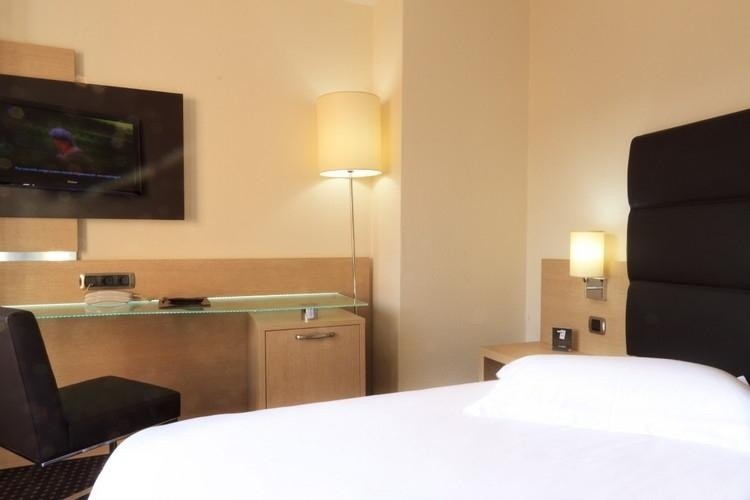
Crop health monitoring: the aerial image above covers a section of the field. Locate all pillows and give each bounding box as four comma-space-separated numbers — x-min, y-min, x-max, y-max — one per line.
495, 353, 749, 448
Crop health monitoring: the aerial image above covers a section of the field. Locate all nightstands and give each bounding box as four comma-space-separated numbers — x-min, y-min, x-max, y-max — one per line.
482, 258, 630, 383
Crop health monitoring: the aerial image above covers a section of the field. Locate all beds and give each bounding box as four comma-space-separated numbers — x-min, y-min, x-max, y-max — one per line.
88, 109, 750, 500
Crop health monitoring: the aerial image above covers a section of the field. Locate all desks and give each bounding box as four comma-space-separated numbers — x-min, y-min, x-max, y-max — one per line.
2, 293, 373, 415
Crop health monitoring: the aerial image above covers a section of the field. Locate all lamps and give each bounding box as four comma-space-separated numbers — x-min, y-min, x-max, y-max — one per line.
312, 89, 387, 317
568, 230, 608, 302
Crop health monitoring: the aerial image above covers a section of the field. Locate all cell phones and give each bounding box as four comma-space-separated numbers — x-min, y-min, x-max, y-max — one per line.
159, 296, 212, 311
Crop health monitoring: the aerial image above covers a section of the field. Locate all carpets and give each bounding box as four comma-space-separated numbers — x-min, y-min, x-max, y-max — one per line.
0, 454, 109, 499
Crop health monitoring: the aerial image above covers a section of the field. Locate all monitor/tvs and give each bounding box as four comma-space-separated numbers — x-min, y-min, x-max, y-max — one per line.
0, 73, 185, 220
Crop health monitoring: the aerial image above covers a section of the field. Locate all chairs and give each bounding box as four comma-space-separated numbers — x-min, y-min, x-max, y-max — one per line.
0, 308, 183, 500
